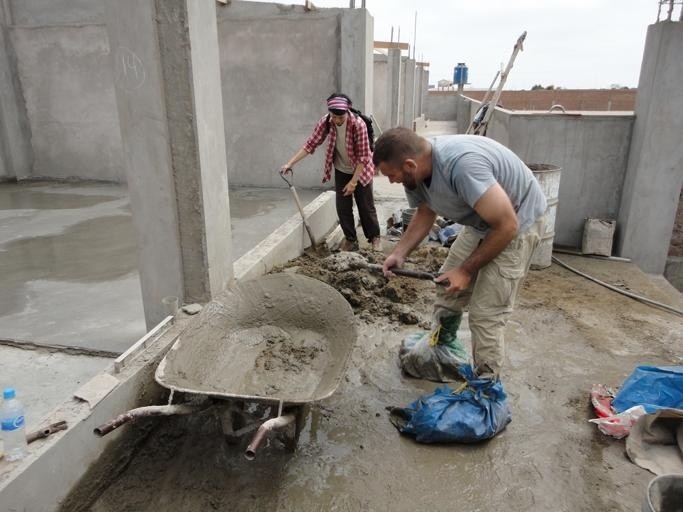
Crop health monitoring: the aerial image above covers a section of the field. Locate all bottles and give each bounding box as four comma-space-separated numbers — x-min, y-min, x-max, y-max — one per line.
0, 387, 30, 461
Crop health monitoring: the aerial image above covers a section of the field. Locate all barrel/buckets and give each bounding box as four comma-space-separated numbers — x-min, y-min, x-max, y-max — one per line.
582, 217, 617, 258
528, 162, 563, 271
401, 209, 428, 248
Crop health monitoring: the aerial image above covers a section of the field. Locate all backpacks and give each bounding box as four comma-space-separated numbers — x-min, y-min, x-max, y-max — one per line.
350, 108, 374, 152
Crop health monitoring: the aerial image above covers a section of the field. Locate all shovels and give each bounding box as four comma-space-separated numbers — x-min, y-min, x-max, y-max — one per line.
327, 251, 451, 287
278, 169, 331, 258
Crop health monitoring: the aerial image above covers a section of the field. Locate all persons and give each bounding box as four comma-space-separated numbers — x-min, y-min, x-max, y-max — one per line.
370, 124, 547, 386
278, 92, 382, 253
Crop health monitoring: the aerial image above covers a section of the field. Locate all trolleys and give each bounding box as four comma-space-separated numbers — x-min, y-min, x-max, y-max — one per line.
90, 268, 361, 464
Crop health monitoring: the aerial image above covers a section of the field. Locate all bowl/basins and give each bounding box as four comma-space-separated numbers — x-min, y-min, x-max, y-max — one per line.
591, 388, 630, 432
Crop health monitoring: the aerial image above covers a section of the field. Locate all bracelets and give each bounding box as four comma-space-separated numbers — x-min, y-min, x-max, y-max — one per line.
349, 181, 357, 187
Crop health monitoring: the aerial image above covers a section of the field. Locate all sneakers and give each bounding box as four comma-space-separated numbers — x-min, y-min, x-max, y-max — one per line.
371, 238, 383, 251
344, 240, 359, 250
